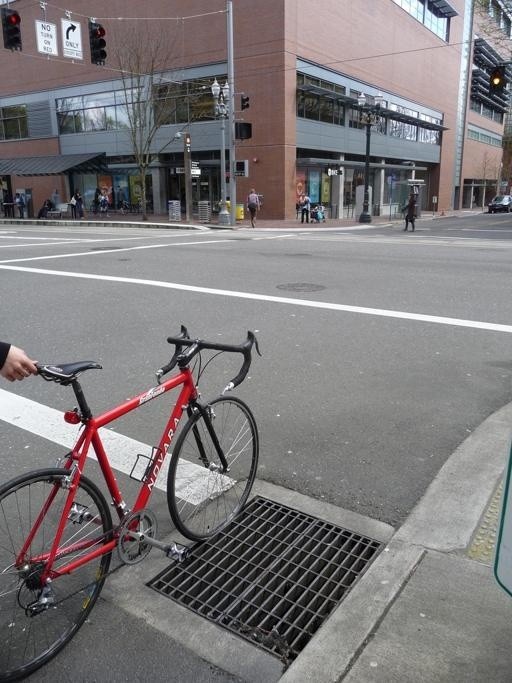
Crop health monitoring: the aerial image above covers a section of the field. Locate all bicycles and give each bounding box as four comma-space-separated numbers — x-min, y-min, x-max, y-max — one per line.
1, 326, 264, 681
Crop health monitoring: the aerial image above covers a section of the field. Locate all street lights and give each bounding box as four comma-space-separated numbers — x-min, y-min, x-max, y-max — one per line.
356, 93, 384, 223
210, 78, 230, 224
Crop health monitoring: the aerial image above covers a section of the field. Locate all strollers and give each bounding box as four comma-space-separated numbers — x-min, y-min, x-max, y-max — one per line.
310, 205, 326, 223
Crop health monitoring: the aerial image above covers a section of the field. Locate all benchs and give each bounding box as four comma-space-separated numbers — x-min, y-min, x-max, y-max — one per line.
35, 202, 71, 219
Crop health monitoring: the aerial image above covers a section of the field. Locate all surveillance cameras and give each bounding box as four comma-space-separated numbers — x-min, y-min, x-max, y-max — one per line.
175, 133, 181, 139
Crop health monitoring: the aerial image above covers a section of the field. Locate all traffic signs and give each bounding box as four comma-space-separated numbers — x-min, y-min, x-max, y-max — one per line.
60, 17, 84, 60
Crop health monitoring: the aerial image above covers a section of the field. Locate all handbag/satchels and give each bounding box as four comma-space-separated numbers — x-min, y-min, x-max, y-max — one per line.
248, 203, 257, 212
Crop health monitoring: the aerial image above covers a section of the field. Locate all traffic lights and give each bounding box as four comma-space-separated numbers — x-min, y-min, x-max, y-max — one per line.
89, 19, 107, 65
0, 7, 20, 46
490, 66, 505, 91
241, 95, 250, 111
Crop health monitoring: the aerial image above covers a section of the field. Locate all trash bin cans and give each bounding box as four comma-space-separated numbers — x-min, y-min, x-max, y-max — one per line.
197, 200, 211, 224
168, 200, 182, 222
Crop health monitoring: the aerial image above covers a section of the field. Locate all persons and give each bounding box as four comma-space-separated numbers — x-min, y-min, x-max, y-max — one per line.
246, 188, 260, 229
299, 193, 311, 224
401, 192, 417, 232
0, 342, 38, 382
3, 187, 126, 219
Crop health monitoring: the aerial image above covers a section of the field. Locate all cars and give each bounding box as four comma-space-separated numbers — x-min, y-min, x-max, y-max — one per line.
488, 195, 512, 212
